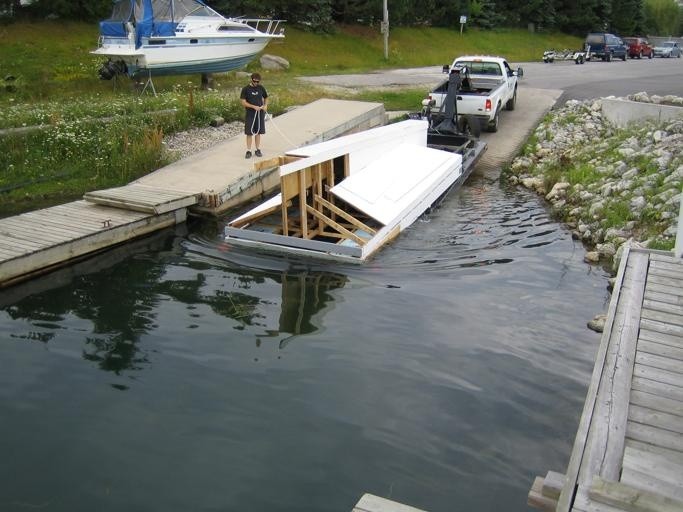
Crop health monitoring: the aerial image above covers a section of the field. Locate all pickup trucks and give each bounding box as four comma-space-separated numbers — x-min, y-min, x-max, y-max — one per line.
418, 54, 523, 132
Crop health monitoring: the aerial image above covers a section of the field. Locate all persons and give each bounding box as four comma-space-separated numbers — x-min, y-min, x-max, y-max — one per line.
239, 71, 270, 160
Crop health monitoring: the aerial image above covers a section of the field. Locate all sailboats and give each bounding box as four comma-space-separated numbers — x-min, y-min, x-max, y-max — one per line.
90, 0, 289, 81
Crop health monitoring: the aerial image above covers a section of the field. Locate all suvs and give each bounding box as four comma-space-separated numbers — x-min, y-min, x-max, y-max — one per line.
584, 33, 628, 61
621, 37, 654, 60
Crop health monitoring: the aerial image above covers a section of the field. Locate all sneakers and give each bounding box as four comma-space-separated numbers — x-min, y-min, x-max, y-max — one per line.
246, 151, 252, 158
255, 149, 262, 157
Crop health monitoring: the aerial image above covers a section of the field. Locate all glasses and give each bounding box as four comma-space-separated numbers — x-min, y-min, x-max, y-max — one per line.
252, 80, 260, 83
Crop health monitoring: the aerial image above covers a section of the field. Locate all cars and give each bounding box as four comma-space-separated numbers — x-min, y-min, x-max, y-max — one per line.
653, 41, 681, 58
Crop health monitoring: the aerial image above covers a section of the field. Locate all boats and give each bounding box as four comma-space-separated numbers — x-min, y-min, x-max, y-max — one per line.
216, 113, 486, 269
